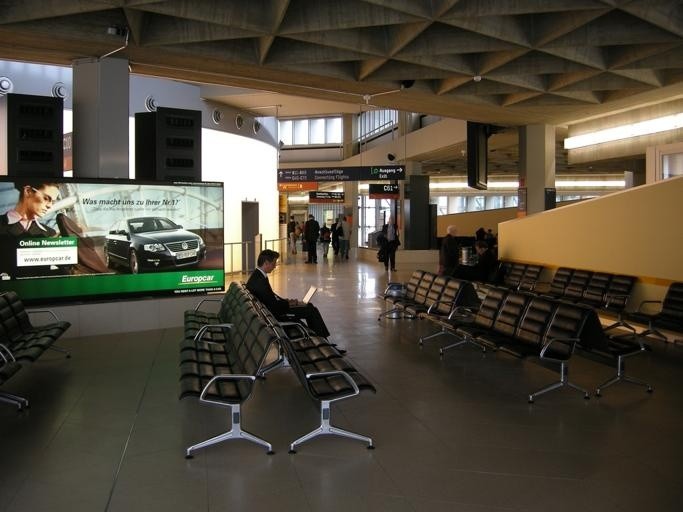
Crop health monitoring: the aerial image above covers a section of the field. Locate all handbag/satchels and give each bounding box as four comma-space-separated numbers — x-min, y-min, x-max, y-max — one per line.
336, 221, 343, 236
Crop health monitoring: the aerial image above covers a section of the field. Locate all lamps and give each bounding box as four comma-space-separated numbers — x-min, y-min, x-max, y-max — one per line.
562, 110, 683, 150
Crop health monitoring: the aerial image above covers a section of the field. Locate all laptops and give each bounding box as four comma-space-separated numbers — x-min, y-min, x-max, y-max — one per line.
291, 285, 317, 307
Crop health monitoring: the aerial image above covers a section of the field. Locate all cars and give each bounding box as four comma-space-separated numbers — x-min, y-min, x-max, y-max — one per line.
103, 216, 208, 273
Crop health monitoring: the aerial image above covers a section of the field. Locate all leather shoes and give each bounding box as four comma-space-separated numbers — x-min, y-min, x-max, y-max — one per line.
390, 268, 397, 272
385, 267, 388, 272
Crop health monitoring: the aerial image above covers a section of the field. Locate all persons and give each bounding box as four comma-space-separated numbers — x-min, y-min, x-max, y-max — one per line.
0, 182, 76, 277
300, 222, 305, 239
320, 222, 332, 257
454, 240, 496, 282
304, 215, 320, 264
297, 221, 301, 239
288, 216, 299, 254
476, 228, 486, 239
336, 215, 352, 259
330, 218, 345, 255
381, 217, 401, 272
438, 225, 459, 277
245, 250, 348, 354
487, 229, 496, 239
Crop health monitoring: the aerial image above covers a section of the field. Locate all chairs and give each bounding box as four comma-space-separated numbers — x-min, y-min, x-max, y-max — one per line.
182, 281, 250, 341
253, 300, 379, 457
238, 280, 316, 339
0, 290, 73, 367
0, 350, 23, 412
484, 283, 653, 396
627, 280, 683, 345
539, 265, 642, 343
177, 300, 284, 460
376, 269, 467, 347
496, 258, 544, 292
